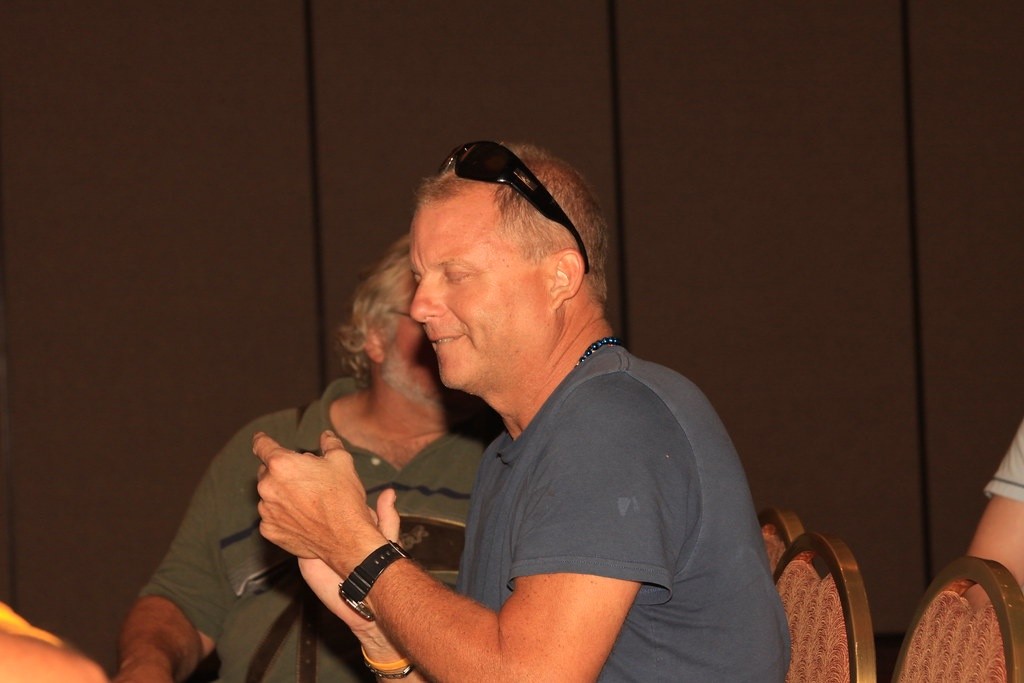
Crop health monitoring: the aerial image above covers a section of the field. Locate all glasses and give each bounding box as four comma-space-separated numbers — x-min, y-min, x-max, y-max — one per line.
437, 141, 591, 276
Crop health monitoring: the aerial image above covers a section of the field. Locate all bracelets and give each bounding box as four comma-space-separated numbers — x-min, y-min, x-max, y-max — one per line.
361, 644, 411, 669
370, 665, 415, 679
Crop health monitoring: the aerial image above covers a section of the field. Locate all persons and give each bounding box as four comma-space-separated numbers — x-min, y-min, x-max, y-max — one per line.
962, 421, 1024, 612
104, 235, 494, 683
0, 601, 110, 683
252, 143, 791, 683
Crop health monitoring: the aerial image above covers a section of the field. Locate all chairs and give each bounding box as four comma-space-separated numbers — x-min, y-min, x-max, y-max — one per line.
755, 504, 1024, 683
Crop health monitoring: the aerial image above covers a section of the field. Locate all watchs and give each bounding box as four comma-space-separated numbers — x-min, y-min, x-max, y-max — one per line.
337, 540, 411, 619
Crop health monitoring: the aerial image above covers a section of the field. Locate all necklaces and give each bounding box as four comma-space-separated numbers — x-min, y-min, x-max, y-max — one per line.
580, 337, 621, 363
